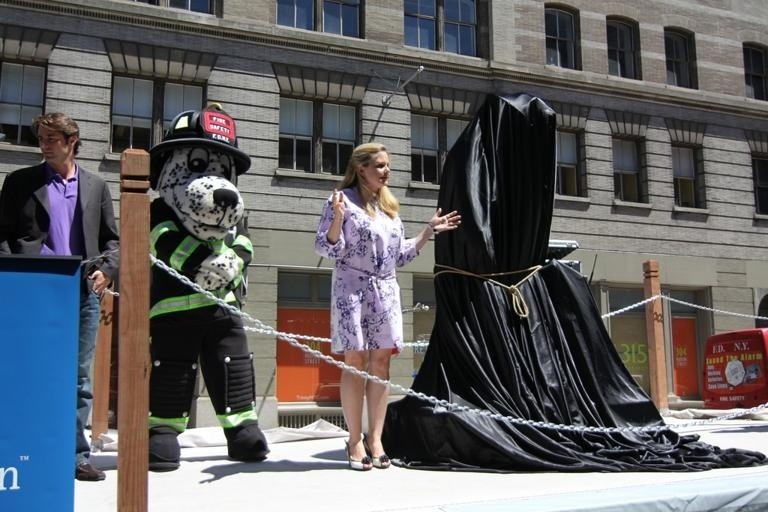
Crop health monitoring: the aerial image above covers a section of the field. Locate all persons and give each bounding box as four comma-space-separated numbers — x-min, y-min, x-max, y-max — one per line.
315, 143, 464, 471
2, 111, 119, 481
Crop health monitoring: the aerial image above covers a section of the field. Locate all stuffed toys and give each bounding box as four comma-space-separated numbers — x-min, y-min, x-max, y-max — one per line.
115, 101, 270, 472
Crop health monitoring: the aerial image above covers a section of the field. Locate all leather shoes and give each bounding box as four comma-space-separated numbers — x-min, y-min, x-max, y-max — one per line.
76, 464, 106, 482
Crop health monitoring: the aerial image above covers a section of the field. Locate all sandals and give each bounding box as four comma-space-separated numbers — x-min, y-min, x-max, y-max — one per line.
363, 436, 391, 469
343, 441, 372, 471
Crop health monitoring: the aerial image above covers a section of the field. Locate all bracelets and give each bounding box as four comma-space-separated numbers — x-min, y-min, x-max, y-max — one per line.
428, 223, 436, 234
